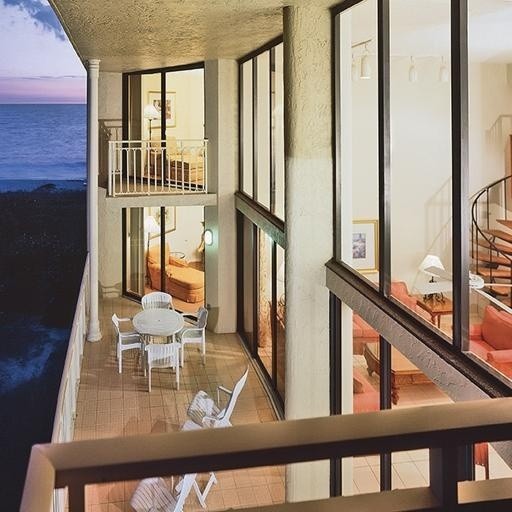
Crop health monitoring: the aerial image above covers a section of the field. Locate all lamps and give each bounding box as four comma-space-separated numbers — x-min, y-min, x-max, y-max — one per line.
419, 255, 446, 303
359, 41, 377, 79
146, 216, 160, 284
439, 57, 451, 83
407, 56, 419, 81
144, 104, 160, 175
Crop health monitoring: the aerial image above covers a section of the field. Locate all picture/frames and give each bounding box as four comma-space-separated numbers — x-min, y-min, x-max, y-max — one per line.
147, 90, 179, 130
352, 220, 380, 275
148, 206, 177, 240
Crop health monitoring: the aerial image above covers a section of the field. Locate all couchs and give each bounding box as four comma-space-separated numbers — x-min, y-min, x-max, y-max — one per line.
351, 280, 418, 354
353, 368, 381, 414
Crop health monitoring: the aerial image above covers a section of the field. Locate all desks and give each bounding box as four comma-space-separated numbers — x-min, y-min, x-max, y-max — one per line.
364, 342, 434, 405
414, 296, 454, 328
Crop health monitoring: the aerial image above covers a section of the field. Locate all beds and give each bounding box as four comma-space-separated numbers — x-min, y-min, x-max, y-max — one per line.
146, 135, 204, 183
145, 242, 204, 303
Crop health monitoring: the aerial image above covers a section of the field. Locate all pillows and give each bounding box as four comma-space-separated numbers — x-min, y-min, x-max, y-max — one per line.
353, 376, 362, 392
480, 305, 512, 350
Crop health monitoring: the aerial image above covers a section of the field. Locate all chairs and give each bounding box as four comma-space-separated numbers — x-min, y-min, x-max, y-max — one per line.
111, 291, 208, 393
452, 302, 512, 380
128, 364, 250, 512
